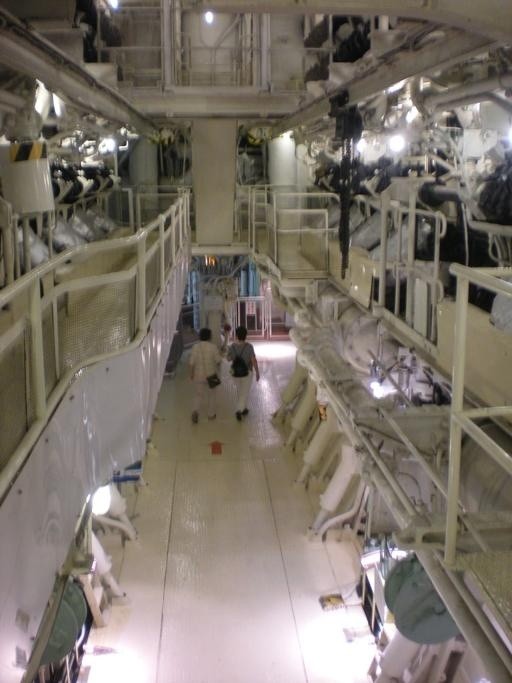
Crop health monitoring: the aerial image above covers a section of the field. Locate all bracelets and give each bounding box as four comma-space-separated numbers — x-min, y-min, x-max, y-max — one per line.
255, 367, 258, 370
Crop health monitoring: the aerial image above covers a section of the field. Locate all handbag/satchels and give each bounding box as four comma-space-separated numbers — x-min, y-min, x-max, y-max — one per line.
206, 374, 221, 388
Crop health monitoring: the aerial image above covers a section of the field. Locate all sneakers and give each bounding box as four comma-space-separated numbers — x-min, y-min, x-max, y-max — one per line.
235, 408, 248, 421
208, 414, 216, 421
192, 411, 198, 424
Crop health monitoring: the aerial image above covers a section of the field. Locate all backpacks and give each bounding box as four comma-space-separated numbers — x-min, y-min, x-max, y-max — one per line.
231, 356, 249, 377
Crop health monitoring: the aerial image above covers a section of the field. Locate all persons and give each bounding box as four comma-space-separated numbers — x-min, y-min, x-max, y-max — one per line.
187, 328, 222, 423
225, 325, 260, 421
